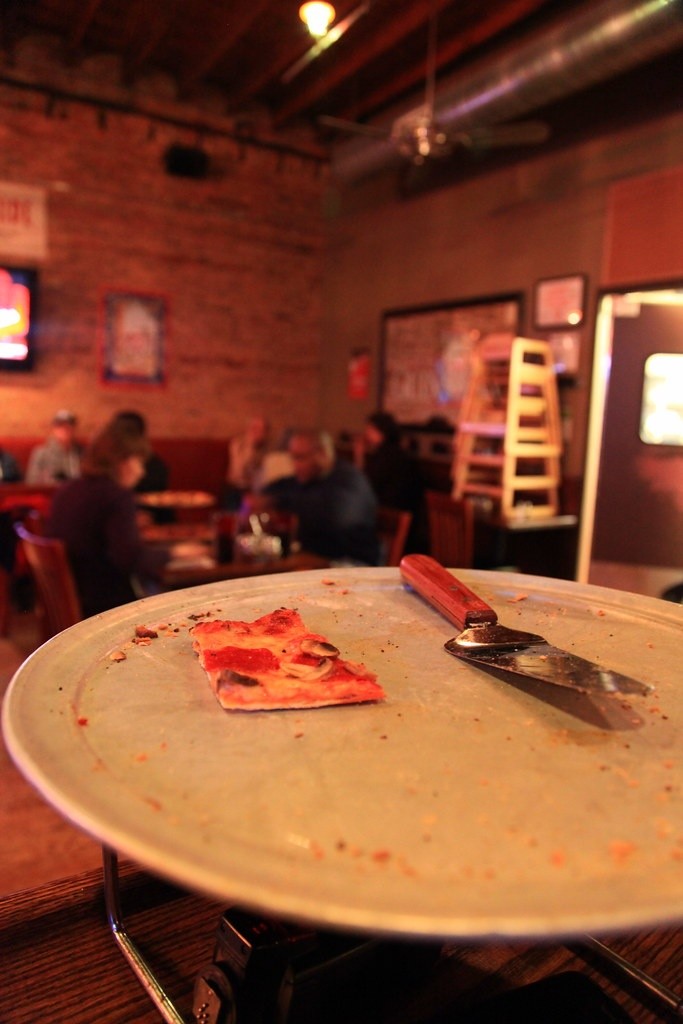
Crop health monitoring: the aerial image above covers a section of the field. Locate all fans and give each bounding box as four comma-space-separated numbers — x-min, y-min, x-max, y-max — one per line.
322, 0, 552, 199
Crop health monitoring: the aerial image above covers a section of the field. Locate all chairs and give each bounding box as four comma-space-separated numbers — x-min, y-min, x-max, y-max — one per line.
377, 505, 410, 567
15, 525, 82, 639
427, 492, 473, 566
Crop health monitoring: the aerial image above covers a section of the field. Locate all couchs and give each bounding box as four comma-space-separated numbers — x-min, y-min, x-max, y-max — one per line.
5, 438, 276, 504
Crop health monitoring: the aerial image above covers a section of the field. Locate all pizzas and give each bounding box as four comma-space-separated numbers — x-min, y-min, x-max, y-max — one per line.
189, 607, 386, 711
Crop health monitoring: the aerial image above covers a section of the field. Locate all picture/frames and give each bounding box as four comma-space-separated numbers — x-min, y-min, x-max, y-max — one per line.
531, 274, 585, 330
378, 293, 525, 431
102, 291, 168, 384
545, 332, 578, 381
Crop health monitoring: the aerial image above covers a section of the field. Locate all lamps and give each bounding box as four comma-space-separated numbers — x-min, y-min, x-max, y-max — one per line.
297, 0, 336, 38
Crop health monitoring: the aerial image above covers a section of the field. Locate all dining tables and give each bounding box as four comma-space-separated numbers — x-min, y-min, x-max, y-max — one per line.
151, 523, 328, 590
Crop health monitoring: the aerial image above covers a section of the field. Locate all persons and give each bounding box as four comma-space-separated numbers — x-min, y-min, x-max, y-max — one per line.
102, 409, 176, 523
290, 433, 376, 570
0, 433, 35, 612
242, 423, 292, 491
255, 431, 321, 536
361, 409, 430, 558
42, 426, 162, 623
24, 409, 107, 483
220, 415, 280, 518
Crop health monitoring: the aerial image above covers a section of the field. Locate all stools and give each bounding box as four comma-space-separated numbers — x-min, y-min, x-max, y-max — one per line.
447, 335, 562, 520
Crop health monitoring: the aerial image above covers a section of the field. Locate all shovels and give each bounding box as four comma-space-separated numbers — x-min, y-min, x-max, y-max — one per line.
401, 553, 657, 701
